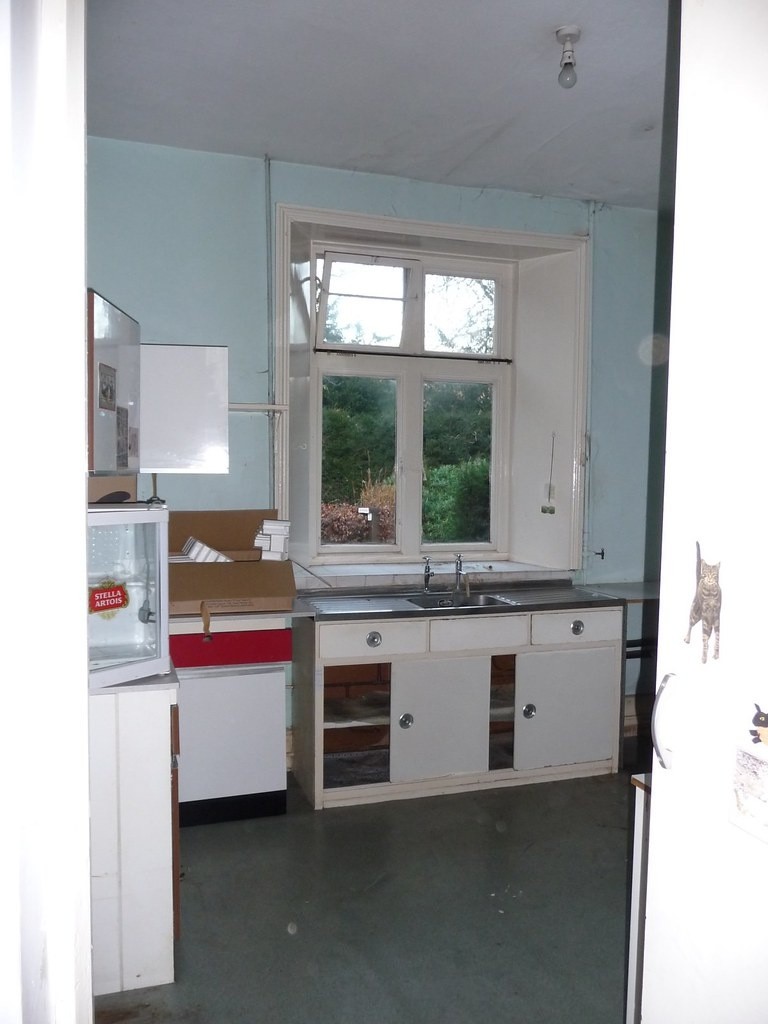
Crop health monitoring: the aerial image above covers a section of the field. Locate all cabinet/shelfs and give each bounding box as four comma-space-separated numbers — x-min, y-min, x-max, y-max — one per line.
89, 659, 181, 997
292, 607, 622, 809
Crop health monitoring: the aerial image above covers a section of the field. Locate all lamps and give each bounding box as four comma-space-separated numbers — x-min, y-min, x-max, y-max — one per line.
557, 27, 580, 88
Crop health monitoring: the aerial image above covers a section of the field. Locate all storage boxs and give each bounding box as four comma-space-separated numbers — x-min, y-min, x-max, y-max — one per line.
87, 502, 170, 688
168, 510, 295, 615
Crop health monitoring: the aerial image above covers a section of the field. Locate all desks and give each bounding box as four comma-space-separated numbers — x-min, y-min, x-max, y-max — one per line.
587, 581, 659, 769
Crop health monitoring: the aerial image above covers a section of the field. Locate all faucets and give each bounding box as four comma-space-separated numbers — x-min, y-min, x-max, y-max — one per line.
452, 551, 471, 599
422, 555, 436, 592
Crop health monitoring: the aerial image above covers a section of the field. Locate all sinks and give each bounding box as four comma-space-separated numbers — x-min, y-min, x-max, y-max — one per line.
405, 592, 514, 609
